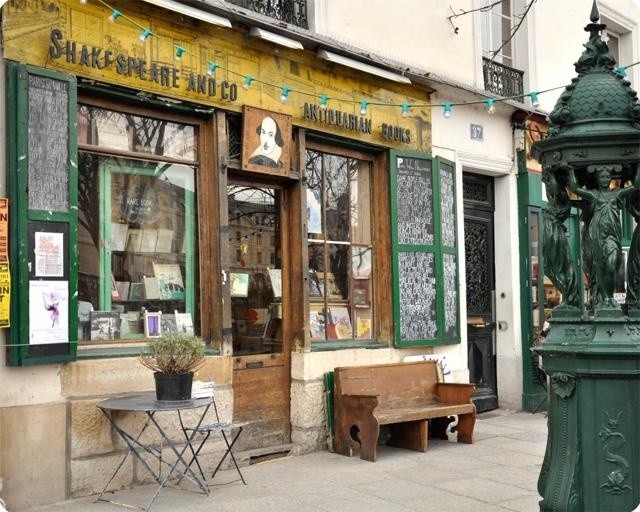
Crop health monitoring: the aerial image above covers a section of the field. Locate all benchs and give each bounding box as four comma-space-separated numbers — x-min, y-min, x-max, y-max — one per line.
333, 360, 479, 460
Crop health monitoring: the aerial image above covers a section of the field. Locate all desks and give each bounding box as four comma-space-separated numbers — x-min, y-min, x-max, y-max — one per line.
99, 395, 220, 512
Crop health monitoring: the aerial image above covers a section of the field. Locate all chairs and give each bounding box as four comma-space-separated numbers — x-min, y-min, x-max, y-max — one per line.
174, 379, 253, 496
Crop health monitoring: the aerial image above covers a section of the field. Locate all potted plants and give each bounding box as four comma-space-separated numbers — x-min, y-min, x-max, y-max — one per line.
139, 329, 209, 399
